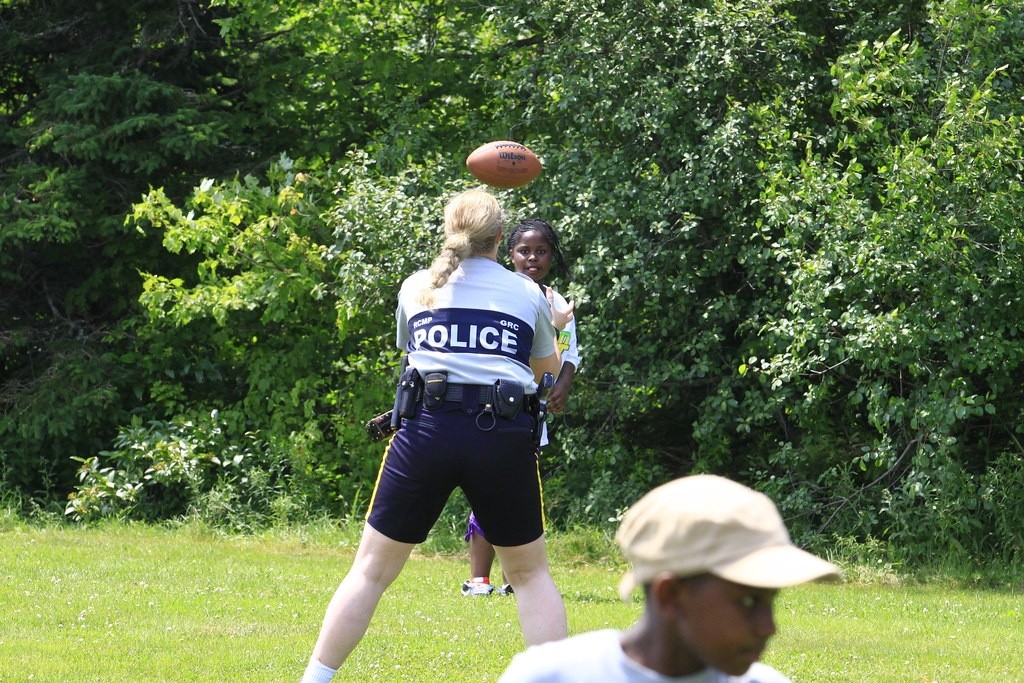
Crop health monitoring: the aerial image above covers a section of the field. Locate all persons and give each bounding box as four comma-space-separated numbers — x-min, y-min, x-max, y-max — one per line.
299, 183, 582, 683
491, 471, 851, 683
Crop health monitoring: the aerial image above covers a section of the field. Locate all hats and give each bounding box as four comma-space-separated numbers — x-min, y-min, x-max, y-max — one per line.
610, 473, 843, 606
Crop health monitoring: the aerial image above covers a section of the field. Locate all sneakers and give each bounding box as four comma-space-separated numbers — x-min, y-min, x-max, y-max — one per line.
459, 575, 514, 599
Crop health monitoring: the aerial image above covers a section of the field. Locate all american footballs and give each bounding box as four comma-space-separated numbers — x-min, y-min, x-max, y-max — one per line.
466, 140, 541, 189
